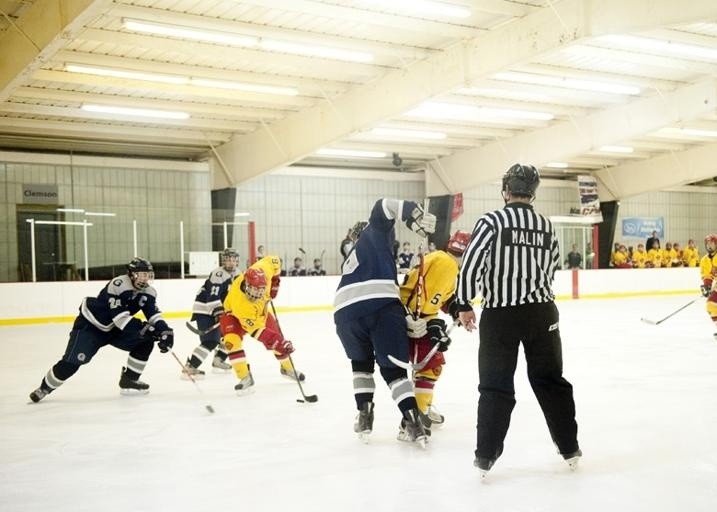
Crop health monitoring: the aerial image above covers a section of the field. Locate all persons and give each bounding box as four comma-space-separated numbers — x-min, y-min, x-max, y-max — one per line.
563, 230, 699, 269
257, 244, 264, 259
29, 256, 175, 404
457, 164, 580, 472
182, 247, 240, 373
699, 235, 717, 329
289, 255, 303, 275
333, 196, 438, 442
307, 259, 324, 274
399, 230, 473, 424
399, 241, 413, 269
218, 254, 305, 389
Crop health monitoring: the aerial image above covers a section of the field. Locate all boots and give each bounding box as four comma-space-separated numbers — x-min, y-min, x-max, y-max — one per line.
234, 372, 255, 390
279, 366, 305, 381
29, 376, 57, 403
404, 407, 425, 441
118, 365, 151, 390
351, 400, 374, 435
182, 358, 205, 374
423, 406, 445, 423
212, 355, 232, 369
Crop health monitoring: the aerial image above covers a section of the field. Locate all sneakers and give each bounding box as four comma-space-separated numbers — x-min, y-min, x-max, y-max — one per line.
560, 447, 582, 463
472, 454, 494, 472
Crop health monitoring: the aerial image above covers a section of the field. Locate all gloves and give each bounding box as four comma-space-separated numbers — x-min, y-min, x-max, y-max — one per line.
700, 284, 712, 297
263, 335, 296, 354
157, 326, 174, 353
448, 296, 467, 327
405, 313, 428, 340
139, 320, 160, 338
270, 276, 280, 298
426, 317, 452, 352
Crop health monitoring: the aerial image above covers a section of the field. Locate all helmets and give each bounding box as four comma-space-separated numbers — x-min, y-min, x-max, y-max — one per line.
244, 268, 268, 302
220, 248, 240, 272
125, 256, 155, 290
704, 233, 717, 254
444, 229, 471, 255
501, 163, 541, 199
350, 221, 370, 242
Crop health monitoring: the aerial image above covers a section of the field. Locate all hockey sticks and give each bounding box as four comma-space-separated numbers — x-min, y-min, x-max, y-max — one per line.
187, 322, 221, 335
167, 347, 215, 414
270, 300, 317, 402
640, 290, 717, 325
387, 303, 475, 370
